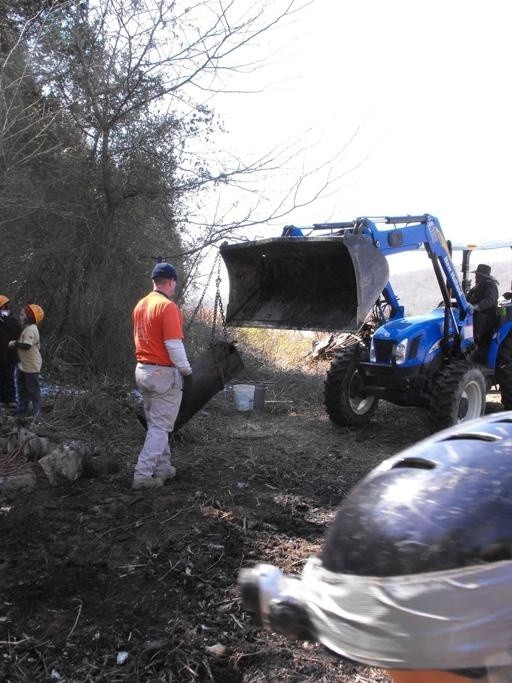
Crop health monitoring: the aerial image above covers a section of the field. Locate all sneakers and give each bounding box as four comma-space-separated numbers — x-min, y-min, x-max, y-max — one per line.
132, 466, 177, 491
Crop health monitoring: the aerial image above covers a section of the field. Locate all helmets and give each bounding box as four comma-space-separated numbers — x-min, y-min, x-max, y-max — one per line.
26, 304, 44, 324
0, 295, 10, 307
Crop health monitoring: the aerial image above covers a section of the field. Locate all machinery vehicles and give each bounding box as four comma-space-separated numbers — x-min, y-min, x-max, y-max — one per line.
219, 213, 512, 435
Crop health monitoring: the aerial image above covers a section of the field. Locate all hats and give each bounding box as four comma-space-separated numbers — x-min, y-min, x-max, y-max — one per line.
151, 262, 177, 281
470, 264, 494, 280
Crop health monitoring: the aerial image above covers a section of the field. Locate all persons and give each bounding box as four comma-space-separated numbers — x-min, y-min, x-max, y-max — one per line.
465, 264, 500, 362
131, 263, 193, 491
0, 295, 44, 416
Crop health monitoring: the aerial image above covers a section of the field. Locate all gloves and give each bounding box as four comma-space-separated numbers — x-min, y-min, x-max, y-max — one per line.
184, 373, 193, 394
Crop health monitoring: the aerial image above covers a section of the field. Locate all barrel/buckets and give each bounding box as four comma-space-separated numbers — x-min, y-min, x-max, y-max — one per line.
232, 385, 256, 412
